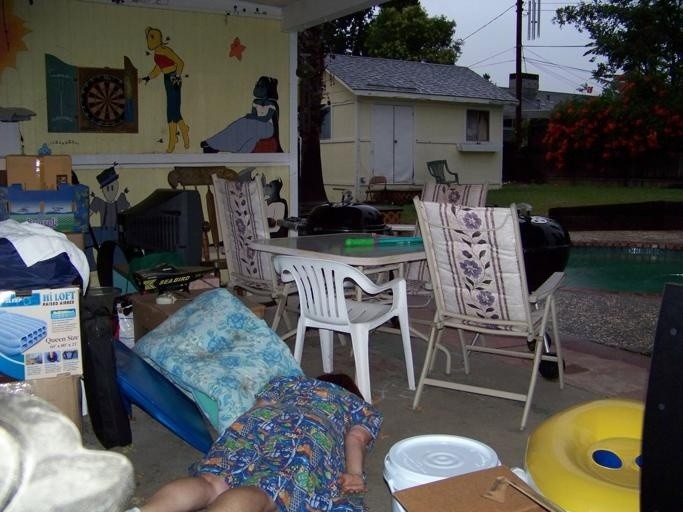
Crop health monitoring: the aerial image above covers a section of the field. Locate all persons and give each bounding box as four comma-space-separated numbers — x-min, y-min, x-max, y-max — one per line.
122, 372, 383, 512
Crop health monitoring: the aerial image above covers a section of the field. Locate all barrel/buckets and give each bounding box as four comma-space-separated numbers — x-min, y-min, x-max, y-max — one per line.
380, 433, 503, 511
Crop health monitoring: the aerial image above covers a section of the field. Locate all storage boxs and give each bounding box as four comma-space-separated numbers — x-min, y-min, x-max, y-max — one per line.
0, 284, 83, 426
6, 153, 90, 232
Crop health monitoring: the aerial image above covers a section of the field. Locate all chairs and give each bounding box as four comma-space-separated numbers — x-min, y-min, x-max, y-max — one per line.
273, 254, 416, 404
384, 183, 488, 327
212, 173, 347, 347
412, 195, 565, 432
